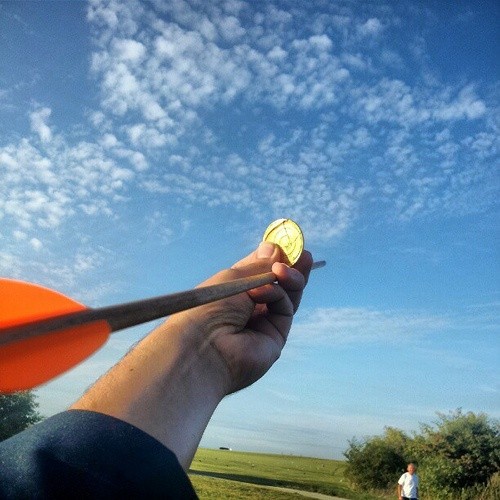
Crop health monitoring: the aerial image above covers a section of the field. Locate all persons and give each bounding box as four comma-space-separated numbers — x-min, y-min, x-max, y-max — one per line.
0, 241, 313, 500
397, 463, 419, 500
